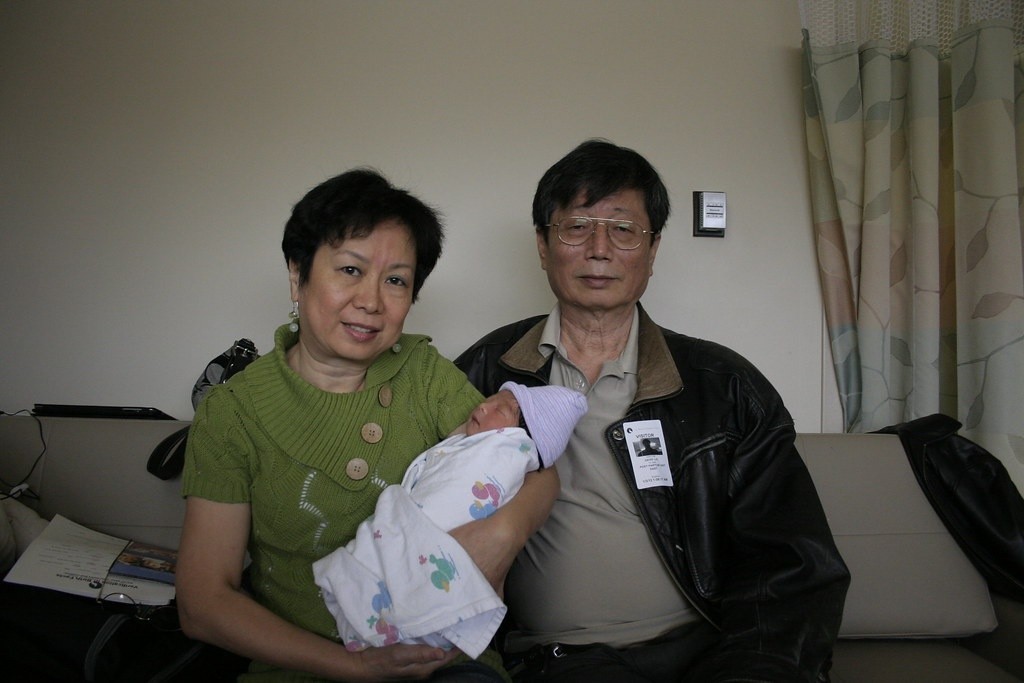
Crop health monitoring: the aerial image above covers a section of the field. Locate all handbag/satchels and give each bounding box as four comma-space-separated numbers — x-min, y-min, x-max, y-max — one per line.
191, 337, 260, 413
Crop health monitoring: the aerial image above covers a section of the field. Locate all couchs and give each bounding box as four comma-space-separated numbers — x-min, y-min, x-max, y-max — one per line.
0, 415, 1024, 683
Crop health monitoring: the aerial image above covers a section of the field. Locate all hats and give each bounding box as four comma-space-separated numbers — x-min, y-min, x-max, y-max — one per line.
498, 382, 588, 469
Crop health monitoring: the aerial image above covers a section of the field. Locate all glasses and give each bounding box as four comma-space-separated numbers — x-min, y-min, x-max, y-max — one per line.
95, 539, 183, 632
542, 216, 660, 250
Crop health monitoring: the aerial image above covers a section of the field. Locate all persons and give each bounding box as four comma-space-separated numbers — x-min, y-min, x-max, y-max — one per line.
119, 554, 142, 564
453, 139, 852, 683
174, 168, 561, 683
638, 439, 658, 456
311, 379, 588, 660
